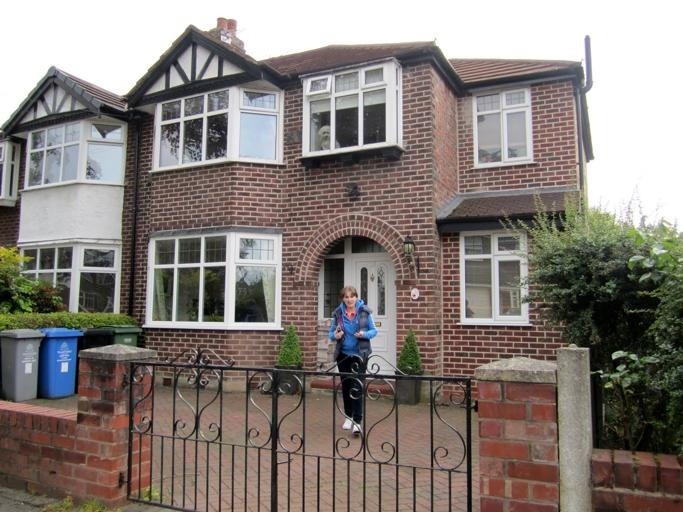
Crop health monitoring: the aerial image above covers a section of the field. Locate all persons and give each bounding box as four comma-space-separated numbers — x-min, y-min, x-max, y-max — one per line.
328, 286, 378, 435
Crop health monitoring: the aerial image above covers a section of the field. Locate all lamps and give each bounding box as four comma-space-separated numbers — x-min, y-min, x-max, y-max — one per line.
403, 236, 417, 260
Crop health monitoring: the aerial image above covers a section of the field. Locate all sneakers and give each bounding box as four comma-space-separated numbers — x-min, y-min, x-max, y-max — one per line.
352, 424, 360, 434
343, 418, 353, 430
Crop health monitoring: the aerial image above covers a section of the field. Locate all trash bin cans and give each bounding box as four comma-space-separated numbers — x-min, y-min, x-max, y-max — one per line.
87, 324, 142, 347
0, 328, 84, 402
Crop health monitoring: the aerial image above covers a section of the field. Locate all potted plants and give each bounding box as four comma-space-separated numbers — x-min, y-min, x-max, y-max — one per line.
276, 324, 304, 394
395, 328, 424, 405
